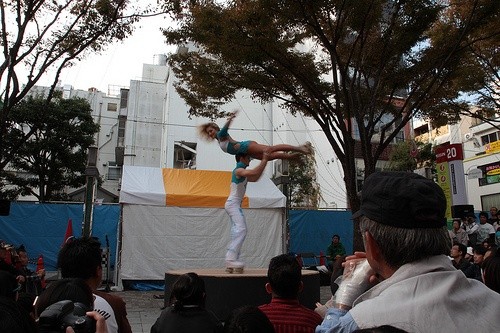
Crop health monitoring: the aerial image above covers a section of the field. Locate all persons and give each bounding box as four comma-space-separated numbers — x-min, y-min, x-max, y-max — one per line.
257, 254, 325, 333
224, 149, 269, 273
315, 171, 500, 333
225, 305, 275, 333
0, 236, 132, 333
198, 110, 311, 160
150, 273, 225, 333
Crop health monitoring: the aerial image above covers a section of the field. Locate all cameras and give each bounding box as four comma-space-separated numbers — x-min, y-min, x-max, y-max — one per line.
37, 300, 96, 333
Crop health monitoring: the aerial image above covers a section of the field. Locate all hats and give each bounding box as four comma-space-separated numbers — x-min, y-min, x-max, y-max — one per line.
351, 170, 448, 229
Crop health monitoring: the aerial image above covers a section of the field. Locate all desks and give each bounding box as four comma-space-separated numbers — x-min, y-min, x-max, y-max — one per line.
163, 269, 321, 309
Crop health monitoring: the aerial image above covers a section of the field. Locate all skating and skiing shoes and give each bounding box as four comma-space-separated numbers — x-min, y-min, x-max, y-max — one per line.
224, 259, 245, 274
